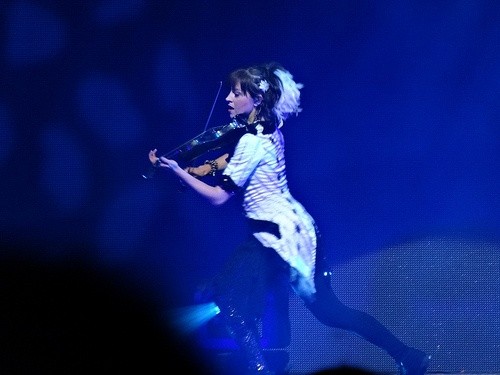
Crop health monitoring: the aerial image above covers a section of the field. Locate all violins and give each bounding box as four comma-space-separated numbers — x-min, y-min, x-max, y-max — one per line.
138, 113, 257, 184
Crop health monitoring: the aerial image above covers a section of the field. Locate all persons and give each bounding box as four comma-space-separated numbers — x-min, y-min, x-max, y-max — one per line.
148, 65, 432, 375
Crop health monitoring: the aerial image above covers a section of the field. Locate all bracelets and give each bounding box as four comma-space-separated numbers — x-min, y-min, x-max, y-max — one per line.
205, 159, 219, 176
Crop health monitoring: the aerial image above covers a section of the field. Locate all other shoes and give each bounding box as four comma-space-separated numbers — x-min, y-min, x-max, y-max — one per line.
395, 347, 431, 375
